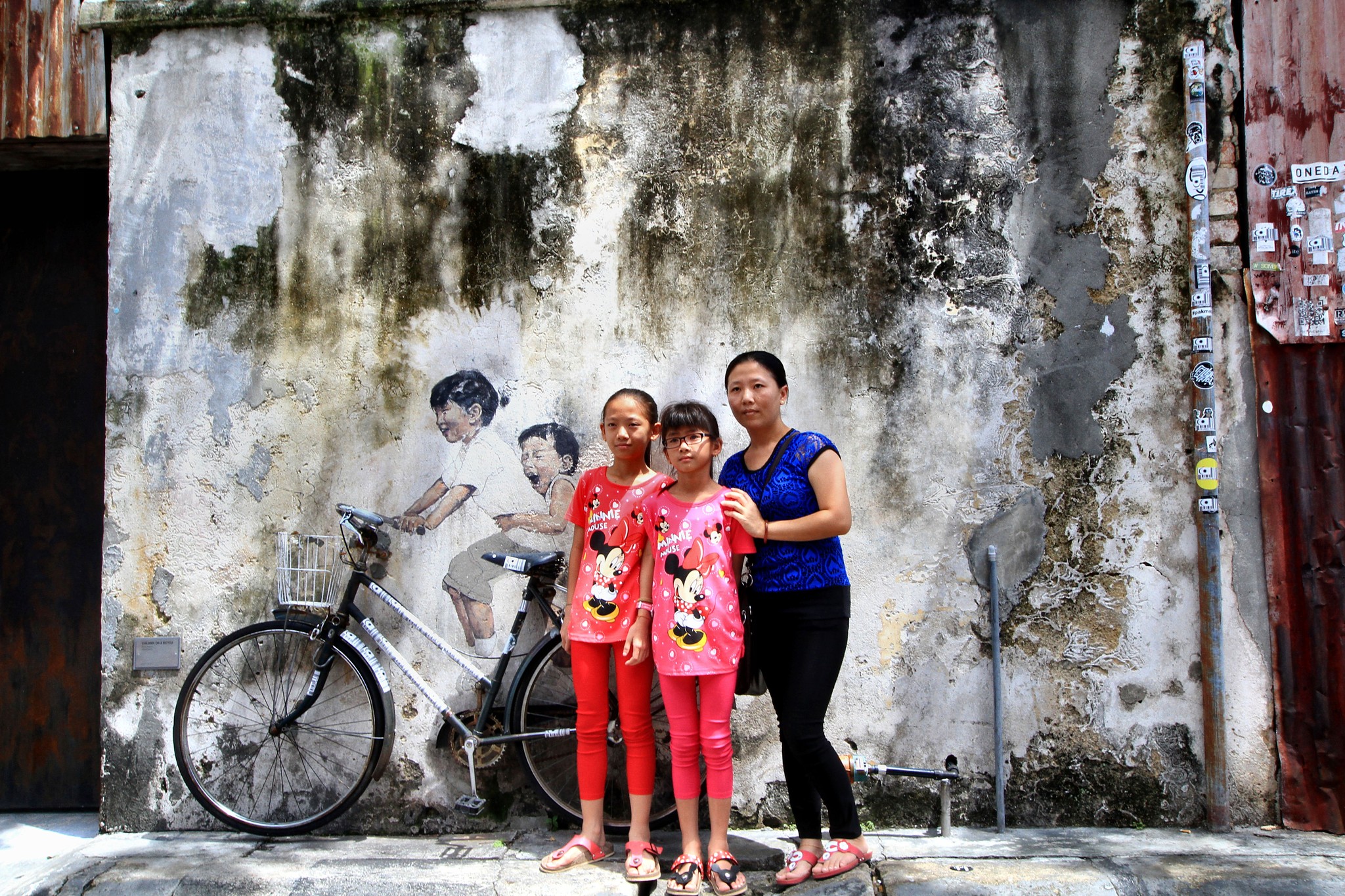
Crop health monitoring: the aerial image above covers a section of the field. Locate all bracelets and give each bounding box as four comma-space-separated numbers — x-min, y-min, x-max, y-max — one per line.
637, 597, 653, 604
565, 603, 572, 606
763, 520, 769, 543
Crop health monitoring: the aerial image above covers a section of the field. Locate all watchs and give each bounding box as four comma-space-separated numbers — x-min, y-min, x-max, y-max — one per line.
635, 601, 655, 617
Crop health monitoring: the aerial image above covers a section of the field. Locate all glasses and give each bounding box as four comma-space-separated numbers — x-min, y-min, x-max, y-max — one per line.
662, 433, 711, 449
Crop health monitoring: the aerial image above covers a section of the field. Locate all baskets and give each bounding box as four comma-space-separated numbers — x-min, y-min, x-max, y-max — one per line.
274, 532, 348, 607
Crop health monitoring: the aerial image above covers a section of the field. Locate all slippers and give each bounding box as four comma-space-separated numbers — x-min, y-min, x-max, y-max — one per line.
812, 839, 872, 879
539, 834, 615, 873
625, 840, 663, 882
775, 850, 818, 885
666, 854, 704, 896
704, 850, 748, 896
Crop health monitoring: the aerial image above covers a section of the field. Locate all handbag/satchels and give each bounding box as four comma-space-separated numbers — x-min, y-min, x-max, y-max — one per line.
734, 581, 768, 696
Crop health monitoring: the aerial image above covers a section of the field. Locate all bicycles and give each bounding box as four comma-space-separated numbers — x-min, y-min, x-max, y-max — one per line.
173, 505, 709, 836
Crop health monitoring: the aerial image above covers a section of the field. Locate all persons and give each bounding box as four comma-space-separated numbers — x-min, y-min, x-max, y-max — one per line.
714, 350, 876, 885
631, 403, 759, 896
538, 389, 681, 882
